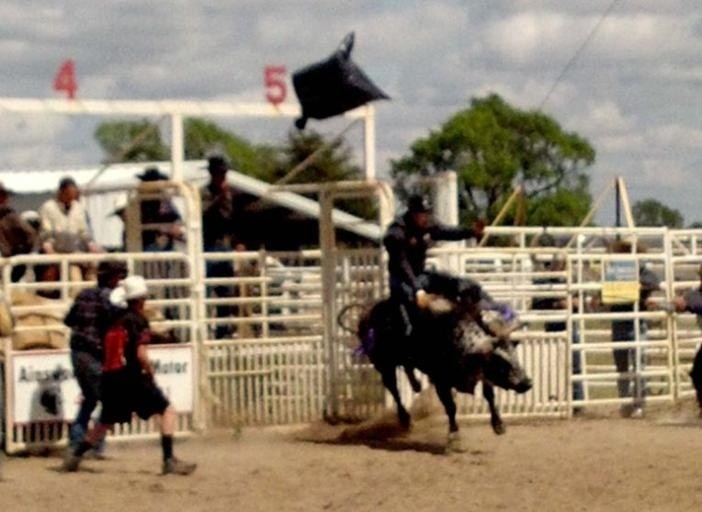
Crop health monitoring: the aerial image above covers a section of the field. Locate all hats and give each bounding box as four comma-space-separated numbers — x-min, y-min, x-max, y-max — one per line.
117, 275, 149, 301
208, 156, 230, 172
138, 168, 169, 181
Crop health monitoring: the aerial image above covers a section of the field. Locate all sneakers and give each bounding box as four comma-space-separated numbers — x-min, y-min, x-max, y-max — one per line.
163, 458, 197, 476
64, 454, 81, 471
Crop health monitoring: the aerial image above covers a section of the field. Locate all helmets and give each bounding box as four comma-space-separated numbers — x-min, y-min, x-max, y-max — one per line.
408, 194, 433, 215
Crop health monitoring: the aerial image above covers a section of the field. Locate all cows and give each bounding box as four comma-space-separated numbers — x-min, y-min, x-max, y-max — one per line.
337, 294, 534, 438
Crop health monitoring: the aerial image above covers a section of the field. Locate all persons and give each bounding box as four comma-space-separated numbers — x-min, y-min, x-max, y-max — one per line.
382, 193, 520, 355
40, 178, 109, 283
645, 264, 701, 419
230, 242, 255, 277
61, 275, 196, 476
529, 260, 592, 413
20, 210, 63, 302
123, 167, 187, 342
202, 154, 240, 341
592, 243, 661, 419
62, 256, 129, 473
0, 182, 37, 283
